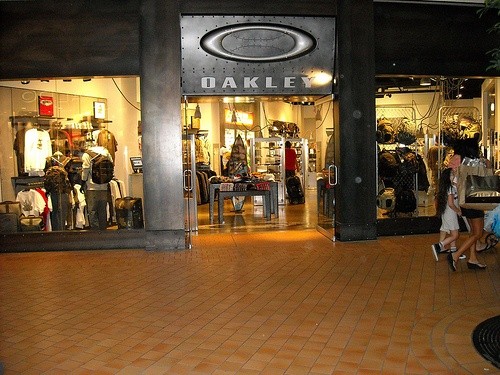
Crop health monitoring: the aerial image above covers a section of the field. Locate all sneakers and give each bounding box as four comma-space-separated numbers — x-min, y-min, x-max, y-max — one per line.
432, 243, 442, 262
450, 249, 467, 260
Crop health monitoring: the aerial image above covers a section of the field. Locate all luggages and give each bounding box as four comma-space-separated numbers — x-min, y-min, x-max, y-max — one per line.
20, 214, 46, 231
0, 201, 22, 233
115, 196, 144, 229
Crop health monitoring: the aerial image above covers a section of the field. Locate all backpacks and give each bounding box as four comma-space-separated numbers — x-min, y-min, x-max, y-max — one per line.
44, 156, 71, 193
87, 150, 114, 184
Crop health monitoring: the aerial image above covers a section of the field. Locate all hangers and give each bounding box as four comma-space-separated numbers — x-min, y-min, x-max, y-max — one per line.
196, 169, 211, 171
18, 182, 45, 194
98, 126, 114, 136
16, 121, 48, 134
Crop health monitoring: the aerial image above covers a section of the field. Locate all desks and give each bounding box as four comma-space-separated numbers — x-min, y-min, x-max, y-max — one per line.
208, 182, 279, 224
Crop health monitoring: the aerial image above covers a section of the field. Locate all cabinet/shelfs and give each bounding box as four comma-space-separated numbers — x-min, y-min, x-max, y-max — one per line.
253, 138, 305, 206
306, 141, 318, 190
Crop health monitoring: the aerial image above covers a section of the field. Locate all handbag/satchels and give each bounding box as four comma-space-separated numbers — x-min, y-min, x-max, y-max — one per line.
458, 164, 500, 211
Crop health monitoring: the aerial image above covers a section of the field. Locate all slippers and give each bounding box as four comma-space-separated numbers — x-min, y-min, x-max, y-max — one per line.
467, 261, 487, 270
448, 253, 457, 273
476, 243, 491, 253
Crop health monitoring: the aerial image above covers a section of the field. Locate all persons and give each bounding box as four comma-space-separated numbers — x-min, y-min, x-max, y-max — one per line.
285, 141, 300, 195
448, 138, 498, 271
80, 145, 114, 231
430, 166, 460, 261
43, 151, 73, 231
426, 135, 445, 201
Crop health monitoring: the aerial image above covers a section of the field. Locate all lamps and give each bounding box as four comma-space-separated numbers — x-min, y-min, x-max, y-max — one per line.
419, 78, 432, 85
